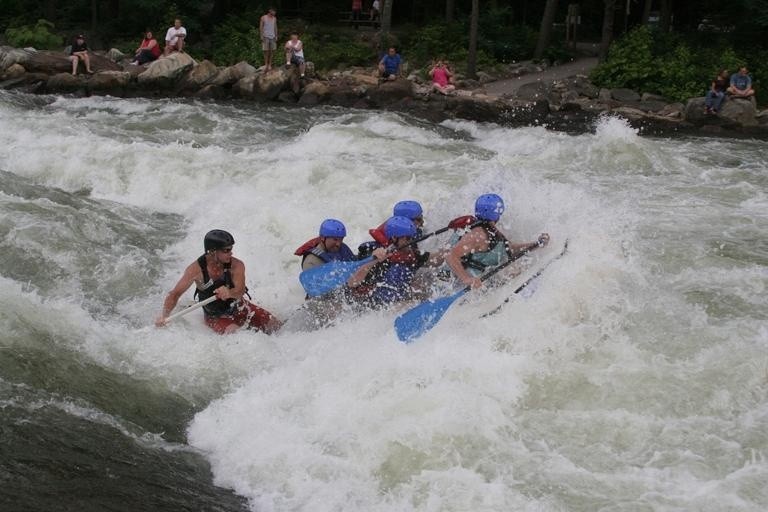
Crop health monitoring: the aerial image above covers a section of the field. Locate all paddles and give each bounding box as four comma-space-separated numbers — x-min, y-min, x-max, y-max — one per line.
300, 227, 452, 295
395, 240, 543, 342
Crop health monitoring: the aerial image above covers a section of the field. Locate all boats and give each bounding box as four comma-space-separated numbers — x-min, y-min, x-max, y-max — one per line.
177, 229, 572, 348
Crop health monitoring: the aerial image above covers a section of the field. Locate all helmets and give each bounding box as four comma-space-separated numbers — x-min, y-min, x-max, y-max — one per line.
320, 219, 346, 238
385, 201, 422, 238
204, 229, 234, 249
475, 194, 504, 222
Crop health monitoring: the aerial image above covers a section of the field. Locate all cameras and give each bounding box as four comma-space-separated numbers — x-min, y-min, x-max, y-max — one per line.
437, 63, 443, 67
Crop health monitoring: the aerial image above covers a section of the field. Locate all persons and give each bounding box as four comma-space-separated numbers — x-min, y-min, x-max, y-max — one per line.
448, 193, 551, 295
428, 58, 456, 97
349, 0, 363, 30
702, 68, 730, 115
378, 46, 404, 81
724, 65, 755, 100
153, 227, 284, 337
294, 217, 357, 304
128, 30, 161, 67
163, 18, 187, 57
283, 31, 305, 78
372, 0, 381, 21
259, 7, 278, 70
69, 34, 95, 76
391, 200, 425, 231
346, 214, 448, 311
